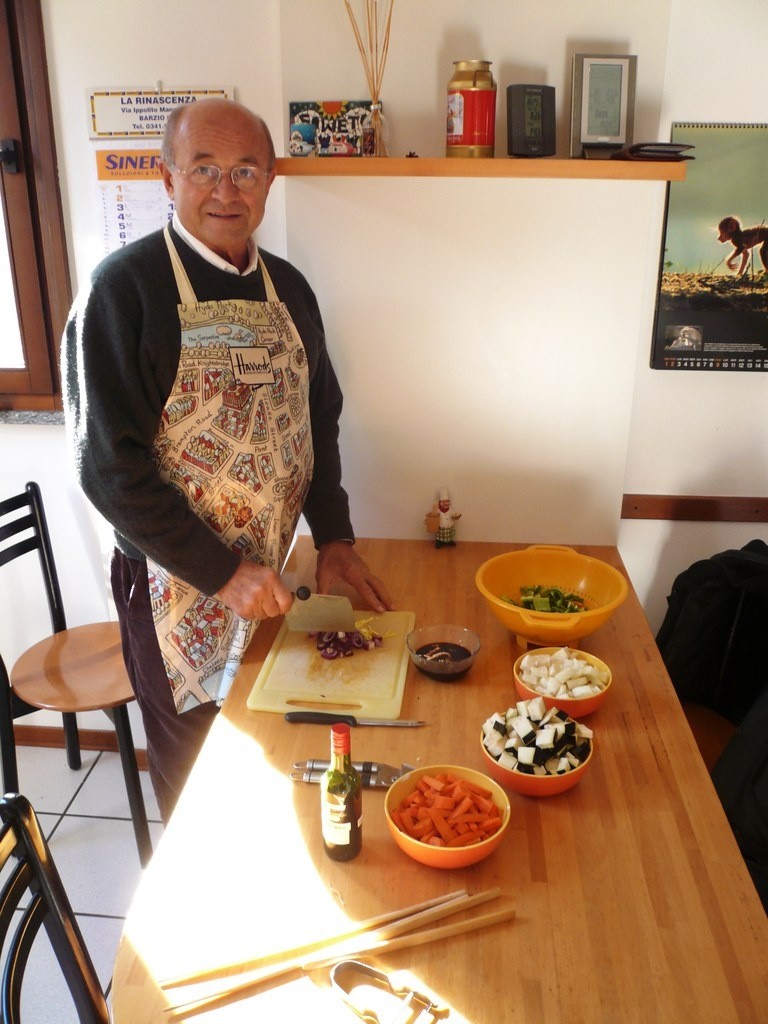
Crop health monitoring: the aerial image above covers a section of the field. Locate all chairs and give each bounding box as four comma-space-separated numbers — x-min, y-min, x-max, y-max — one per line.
0, 790, 111, 1024
669, 554, 768, 774
0, 480, 155, 866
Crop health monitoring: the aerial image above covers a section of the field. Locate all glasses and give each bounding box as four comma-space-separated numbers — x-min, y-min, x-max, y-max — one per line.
170, 161, 270, 193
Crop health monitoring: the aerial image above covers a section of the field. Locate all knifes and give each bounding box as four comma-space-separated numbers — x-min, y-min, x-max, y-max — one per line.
284, 711, 425, 729
285, 590, 355, 632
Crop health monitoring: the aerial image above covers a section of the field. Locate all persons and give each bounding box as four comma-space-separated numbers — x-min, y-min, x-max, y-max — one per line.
59, 97, 397, 832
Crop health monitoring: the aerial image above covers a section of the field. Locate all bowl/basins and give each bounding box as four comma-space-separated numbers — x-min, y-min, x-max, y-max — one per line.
384, 765, 511, 870
475, 544, 629, 645
511, 647, 613, 720
480, 699, 594, 797
406, 623, 482, 680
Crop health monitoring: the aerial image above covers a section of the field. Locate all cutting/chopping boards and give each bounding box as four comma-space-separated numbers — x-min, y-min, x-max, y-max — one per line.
246, 609, 416, 720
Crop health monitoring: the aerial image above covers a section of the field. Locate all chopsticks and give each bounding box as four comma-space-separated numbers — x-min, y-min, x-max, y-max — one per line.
161, 889, 468, 1013
160, 886, 516, 1024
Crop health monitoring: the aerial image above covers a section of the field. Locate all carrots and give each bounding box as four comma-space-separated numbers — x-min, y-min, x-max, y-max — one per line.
391, 773, 502, 847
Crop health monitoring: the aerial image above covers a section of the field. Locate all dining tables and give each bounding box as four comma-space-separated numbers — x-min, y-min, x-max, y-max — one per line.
113, 536, 767, 1024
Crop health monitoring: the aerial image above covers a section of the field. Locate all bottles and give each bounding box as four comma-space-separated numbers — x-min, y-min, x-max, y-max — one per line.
320, 724, 364, 862
